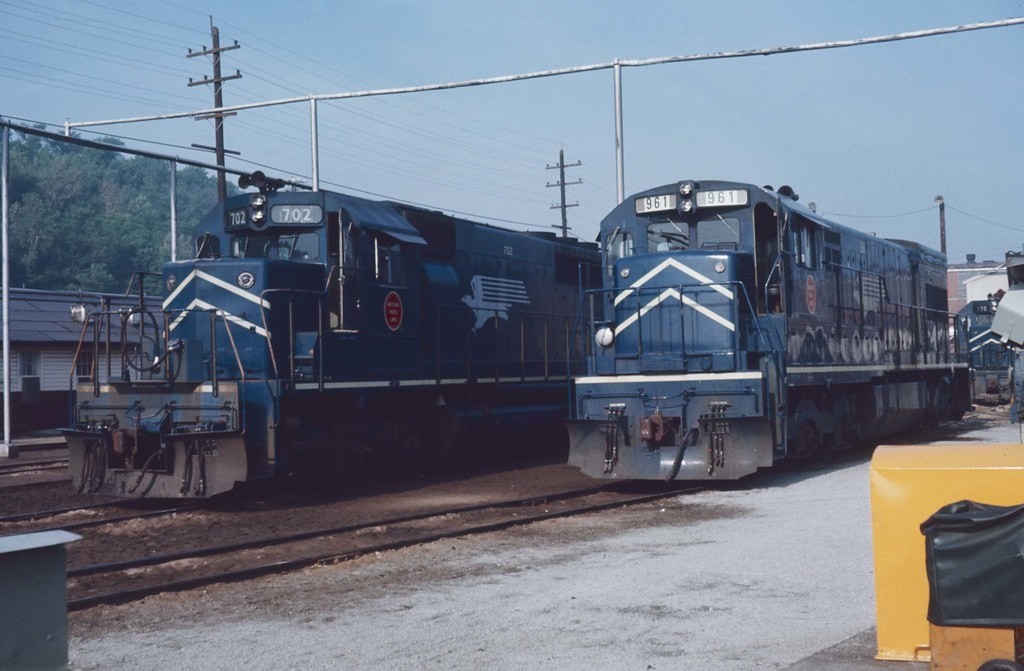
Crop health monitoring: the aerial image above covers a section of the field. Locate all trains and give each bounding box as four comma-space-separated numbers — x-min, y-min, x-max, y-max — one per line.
56, 169, 610, 505
951, 290, 1019, 403
564, 179, 974, 483
